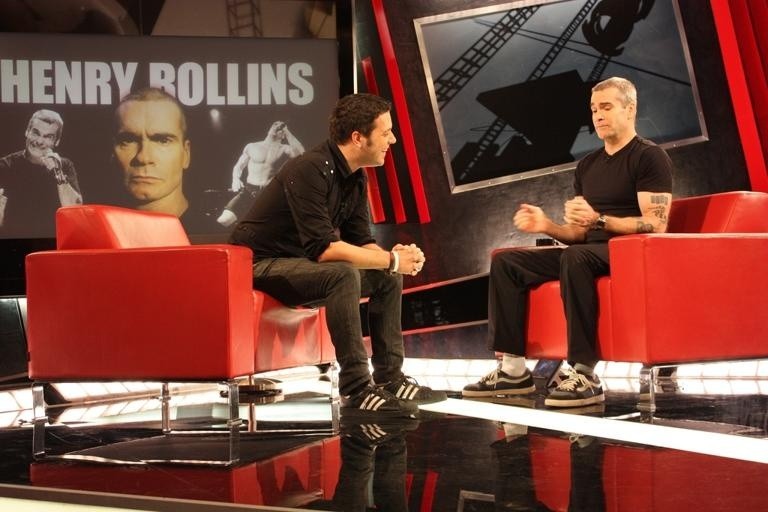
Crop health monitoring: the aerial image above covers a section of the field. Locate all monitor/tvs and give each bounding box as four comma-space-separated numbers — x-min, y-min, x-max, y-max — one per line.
0, 0, 347, 248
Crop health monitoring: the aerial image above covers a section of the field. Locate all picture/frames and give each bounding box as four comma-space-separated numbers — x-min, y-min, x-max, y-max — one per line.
411, 0, 712, 197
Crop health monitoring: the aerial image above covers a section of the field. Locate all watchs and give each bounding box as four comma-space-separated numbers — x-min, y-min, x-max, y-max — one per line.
595, 211, 606, 232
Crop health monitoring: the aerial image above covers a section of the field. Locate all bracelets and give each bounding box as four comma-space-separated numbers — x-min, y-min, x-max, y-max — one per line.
384, 251, 399, 276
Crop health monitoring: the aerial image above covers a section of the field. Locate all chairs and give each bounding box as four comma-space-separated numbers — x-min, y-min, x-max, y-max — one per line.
21, 203, 346, 425
495, 421, 768, 512
27, 421, 345, 510
489, 188, 768, 418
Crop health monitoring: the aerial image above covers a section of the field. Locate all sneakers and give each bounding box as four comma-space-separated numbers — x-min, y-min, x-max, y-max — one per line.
544, 370, 606, 408
340, 415, 420, 449
462, 370, 536, 398
378, 375, 449, 406
339, 384, 417, 418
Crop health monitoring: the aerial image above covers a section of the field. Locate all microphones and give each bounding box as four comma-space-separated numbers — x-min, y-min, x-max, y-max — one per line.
274, 120, 287, 133
44, 146, 61, 183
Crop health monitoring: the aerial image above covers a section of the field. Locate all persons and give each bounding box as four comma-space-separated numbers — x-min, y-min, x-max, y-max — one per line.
217, 119, 305, 228
461, 76, 673, 407
228, 93, 448, 420
0, 110, 82, 240
332, 420, 422, 511
489, 422, 603, 511
109, 86, 228, 244
